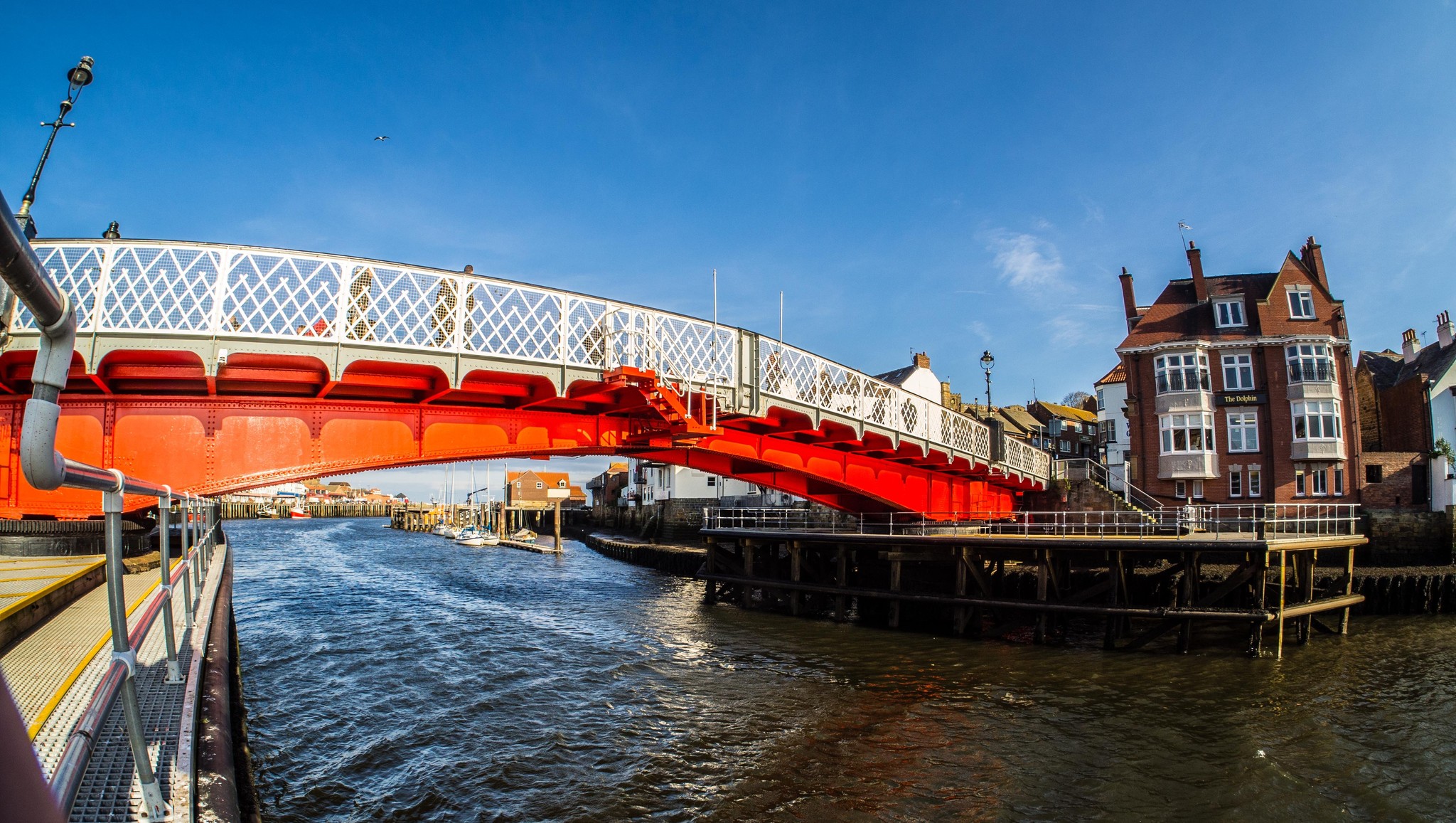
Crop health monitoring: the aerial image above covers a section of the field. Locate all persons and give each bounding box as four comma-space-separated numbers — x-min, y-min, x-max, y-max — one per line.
766, 350, 787, 395
296, 324, 307, 336
227, 316, 242, 333
346, 264, 376, 341
427, 264, 475, 352
583, 317, 605, 367
809, 363, 917, 434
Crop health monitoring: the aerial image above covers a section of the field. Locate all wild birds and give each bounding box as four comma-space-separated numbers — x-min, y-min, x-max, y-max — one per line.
374, 136, 390, 141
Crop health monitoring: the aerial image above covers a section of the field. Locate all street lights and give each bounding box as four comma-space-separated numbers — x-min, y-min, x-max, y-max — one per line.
6, 53, 98, 235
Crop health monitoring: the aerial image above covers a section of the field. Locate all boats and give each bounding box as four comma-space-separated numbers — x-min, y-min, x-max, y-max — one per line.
453, 462, 483, 546
431, 481, 447, 535
491, 463, 509, 540
474, 460, 498, 545
509, 470, 538, 544
443, 461, 463, 539
